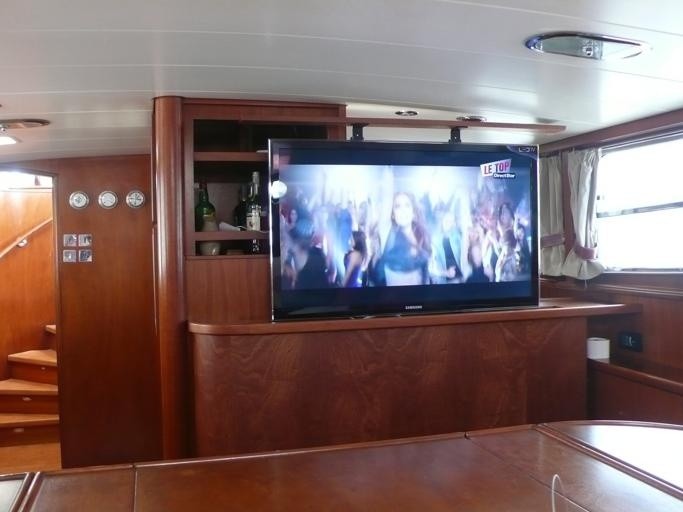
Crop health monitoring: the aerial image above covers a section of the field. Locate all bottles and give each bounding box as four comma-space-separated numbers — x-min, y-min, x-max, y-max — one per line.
196, 166, 271, 257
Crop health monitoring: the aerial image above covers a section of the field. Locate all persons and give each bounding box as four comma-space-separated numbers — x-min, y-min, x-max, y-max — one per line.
279, 177, 532, 290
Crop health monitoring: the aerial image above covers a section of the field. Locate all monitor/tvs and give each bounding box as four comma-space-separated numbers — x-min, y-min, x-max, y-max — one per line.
267, 136, 540, 322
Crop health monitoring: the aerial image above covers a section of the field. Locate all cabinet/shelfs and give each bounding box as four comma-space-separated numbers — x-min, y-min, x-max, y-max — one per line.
181, 105, 335, 257
